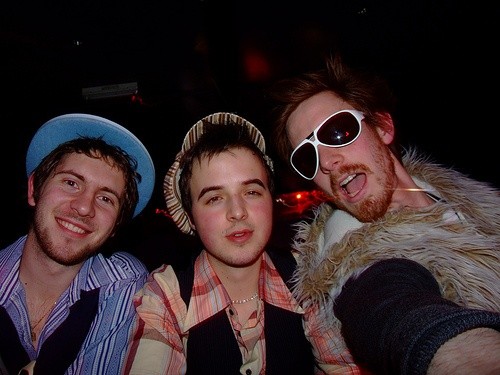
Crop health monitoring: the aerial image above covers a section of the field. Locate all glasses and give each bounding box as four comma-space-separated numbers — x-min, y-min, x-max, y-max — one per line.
289, 109, 367, 182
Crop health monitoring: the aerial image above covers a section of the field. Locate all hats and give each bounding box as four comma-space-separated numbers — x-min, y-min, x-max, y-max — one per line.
163, 112, 275, 239
25, 112, 156, 220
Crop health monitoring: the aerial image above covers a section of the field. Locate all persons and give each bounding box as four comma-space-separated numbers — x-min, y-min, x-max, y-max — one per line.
121, 112, 365, 375
0, 113, 155, 375
272, 63, 500, 375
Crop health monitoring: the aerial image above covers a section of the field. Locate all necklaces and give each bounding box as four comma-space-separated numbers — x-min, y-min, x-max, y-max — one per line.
28, 298, 58, 341
229, 291, 259, 306
